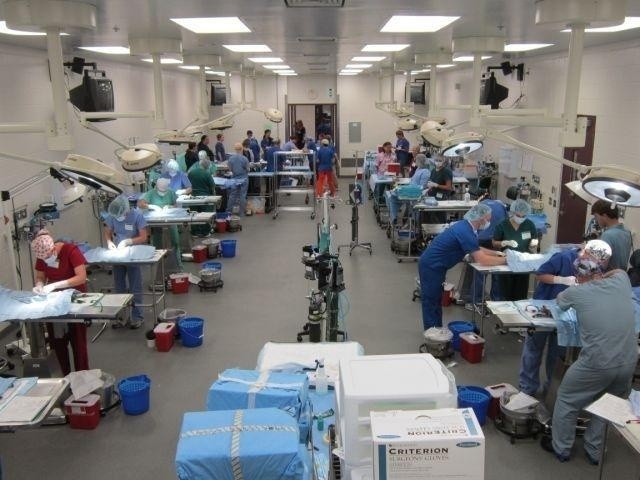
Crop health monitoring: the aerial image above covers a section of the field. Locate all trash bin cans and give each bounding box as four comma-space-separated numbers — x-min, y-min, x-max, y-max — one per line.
423, 327, 453, 357
399, 239, 409, 255
159, 308, 187, 339
90, 369, 116, 415
200, 271, 221, 292
499, 390, 539, 438
201, 238, 221, 259
226, 215, 242, 232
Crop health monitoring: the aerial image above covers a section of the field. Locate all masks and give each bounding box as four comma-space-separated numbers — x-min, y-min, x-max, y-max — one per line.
115, 212, 127, 222
478, 218, 490, 230
156, 190, 167, 197
434, 160, 444, 167
512, 214, 527, 225
43, 247, 57, 264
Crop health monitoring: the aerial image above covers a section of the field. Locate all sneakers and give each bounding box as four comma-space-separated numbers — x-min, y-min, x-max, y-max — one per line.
540, 435, 570, 463
127, 317, 144, 329
112, 322, 124, 329
584, 449, 599, 466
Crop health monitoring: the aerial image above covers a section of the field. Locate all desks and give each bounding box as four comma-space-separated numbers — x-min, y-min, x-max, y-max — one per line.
466, 251, 639, 335
600, 386, 640, 480
61, 237, 167, 327
143, 205, 217, 256
363, 153, 551, 264
0, 377, 73, 432
178, 151, 315, 212
299, 386, 342, 480
0, 287, 134, 377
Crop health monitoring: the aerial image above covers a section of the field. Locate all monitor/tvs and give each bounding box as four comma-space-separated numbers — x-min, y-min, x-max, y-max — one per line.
479, 78, 508, 105
69, 78, 114, 121
211, 82, 226, 105
405, 82, 425, 104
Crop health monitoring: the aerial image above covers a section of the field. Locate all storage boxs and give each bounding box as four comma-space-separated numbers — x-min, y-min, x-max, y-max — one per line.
484, 383, 519, 420
261, 341, 362, 382
337, 350, 456, 472
442, 283, 455, 307
370, 407, 486, 479
192, 245, 207, 263
215, 219, 227, 233
174, 410, 301, 479
64, 393, 102, 430
154, 321, 176, 352
170, 273, 189, 294
206, 367, 315, 442
460, 332, 485, 363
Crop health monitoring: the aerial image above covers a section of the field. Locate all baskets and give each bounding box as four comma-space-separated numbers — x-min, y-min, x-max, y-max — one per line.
180, 317, 203, 329
451, 322, 474, 333
457, 385, 489, 405
119, 378, 147, 393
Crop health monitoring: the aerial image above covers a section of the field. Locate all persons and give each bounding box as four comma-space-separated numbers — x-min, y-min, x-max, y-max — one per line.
103, 196, 149, 330
136, 114, 340, 272
31, 229, 88, 376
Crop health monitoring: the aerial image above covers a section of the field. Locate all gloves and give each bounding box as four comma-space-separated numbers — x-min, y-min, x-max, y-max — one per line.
529, 238, 539, 247
